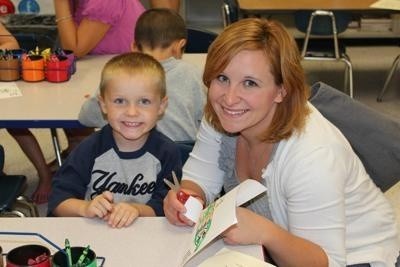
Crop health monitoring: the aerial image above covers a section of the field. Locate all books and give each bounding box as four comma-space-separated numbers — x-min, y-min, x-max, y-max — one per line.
347, 14, 393, 32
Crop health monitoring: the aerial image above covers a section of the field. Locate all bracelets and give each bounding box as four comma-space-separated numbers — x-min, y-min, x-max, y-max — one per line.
55, 14, 72, 24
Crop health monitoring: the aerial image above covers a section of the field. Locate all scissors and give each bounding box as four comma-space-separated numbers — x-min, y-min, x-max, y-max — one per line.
163, 170, 206, 227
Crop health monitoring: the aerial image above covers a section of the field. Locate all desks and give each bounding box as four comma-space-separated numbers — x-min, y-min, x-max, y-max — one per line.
0, 216, 263, 267
0, 52, 206, 170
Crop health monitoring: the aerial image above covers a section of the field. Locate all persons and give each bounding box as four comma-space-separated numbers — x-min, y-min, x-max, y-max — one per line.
44, 52, 182, 229
149, 0, 181, 14
78, 7, 207, 142
163, 17, 399, 267
0, 23, 53, 205
48, 0, 147, 175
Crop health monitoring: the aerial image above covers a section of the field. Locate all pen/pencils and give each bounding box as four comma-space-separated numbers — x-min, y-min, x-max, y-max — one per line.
0, 46, 66, 62
65, 239, 90, 267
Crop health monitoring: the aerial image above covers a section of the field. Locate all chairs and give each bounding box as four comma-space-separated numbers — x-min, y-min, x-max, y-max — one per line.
220, 3, 238, 29
301, 8, 353, 100
